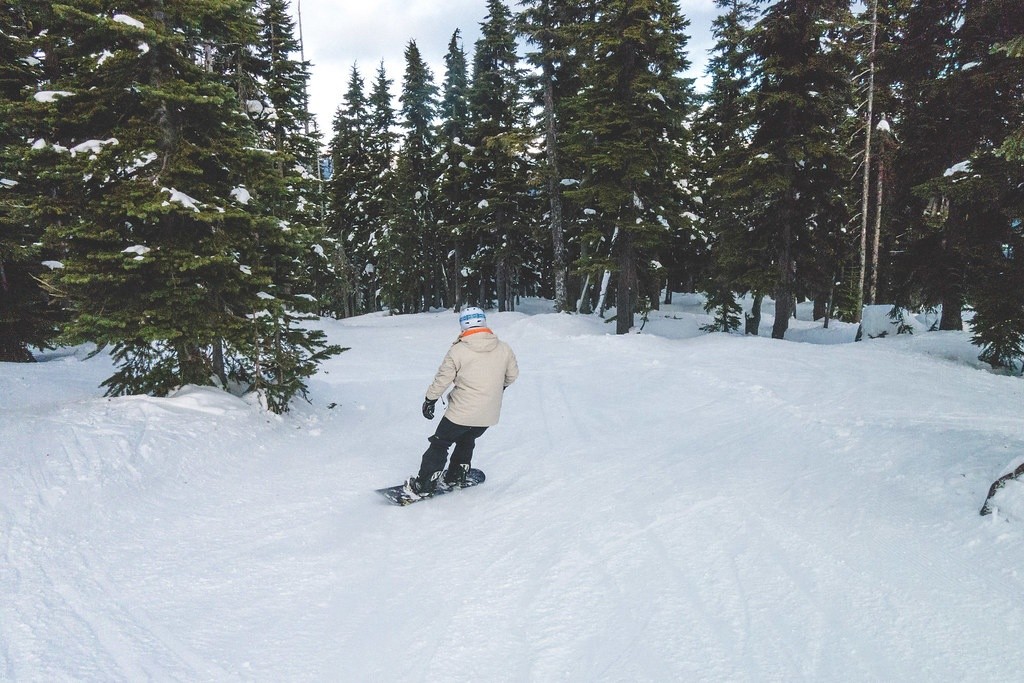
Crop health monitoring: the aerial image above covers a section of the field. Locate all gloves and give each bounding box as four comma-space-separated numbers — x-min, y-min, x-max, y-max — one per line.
422, 396, 438, 419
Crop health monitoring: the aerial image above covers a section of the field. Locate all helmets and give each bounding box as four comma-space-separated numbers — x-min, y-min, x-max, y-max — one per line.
459, 306, 486, 332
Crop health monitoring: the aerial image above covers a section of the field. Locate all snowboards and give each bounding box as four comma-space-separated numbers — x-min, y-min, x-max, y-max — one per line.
375, 467, 485, 509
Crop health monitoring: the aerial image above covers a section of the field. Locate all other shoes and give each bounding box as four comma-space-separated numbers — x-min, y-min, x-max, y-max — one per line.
406, 477, 431, 500
433, 470, 463, 492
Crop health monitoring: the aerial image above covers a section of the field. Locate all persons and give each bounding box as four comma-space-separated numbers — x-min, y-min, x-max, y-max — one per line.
410, 307, 519, 496
